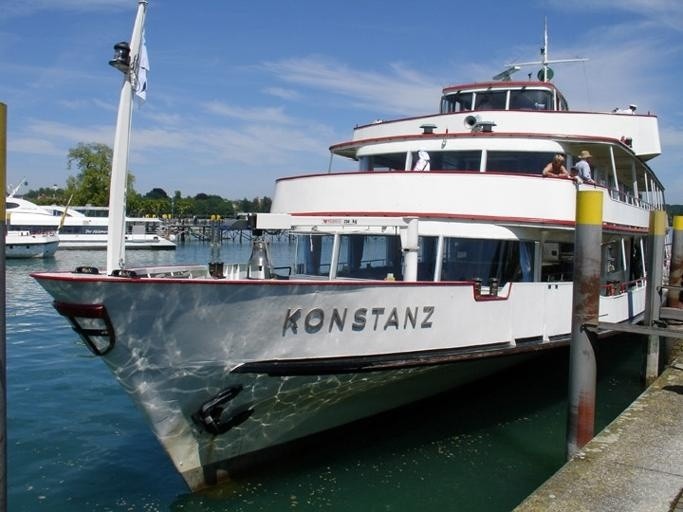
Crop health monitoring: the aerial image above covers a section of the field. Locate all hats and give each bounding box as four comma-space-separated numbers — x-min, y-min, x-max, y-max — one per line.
577, 150, 593, 158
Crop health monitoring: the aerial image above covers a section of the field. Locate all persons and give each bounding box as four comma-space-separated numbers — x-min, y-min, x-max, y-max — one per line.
412, 150, 432, 171
573, 150, 597, 185
541, 154, 569, 178
623, 103, 637, 114
568, 166, 582, 184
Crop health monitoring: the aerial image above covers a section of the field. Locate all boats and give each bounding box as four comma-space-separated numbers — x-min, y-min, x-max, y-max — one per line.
5, 230, 60, 258
27, 0, 668, 494
